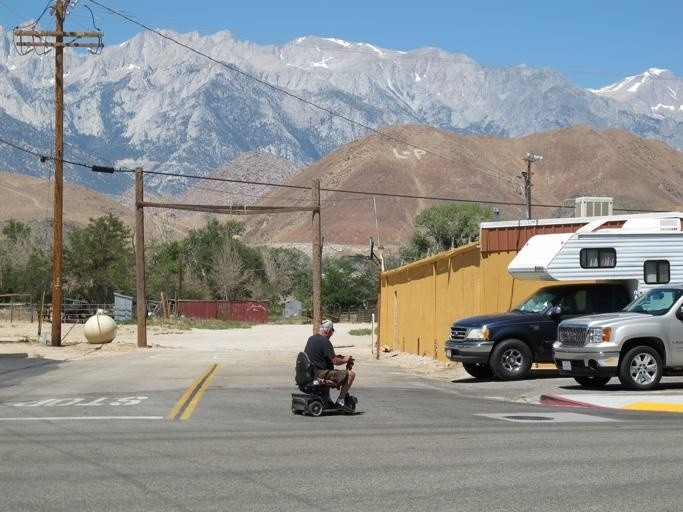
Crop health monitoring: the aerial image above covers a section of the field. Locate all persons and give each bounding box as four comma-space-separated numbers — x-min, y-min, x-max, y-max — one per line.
305, 318, 356, 413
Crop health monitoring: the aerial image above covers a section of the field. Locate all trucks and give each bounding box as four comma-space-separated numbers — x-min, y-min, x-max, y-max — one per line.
443, 213, 683, 379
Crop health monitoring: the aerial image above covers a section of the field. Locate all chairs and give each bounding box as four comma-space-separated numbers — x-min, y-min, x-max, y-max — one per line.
295, 350, 336, 394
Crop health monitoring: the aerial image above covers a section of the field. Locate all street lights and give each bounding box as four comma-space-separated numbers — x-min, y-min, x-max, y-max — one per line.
521, 149, 542, 217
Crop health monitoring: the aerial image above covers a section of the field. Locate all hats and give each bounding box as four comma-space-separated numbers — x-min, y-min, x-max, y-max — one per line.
319, 320, 335, 332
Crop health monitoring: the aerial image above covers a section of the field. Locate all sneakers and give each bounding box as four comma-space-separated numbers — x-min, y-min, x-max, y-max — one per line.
335, 402, 354, 413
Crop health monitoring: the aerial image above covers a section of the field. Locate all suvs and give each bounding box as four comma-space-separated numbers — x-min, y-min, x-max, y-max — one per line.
553, 281, 682, 389
